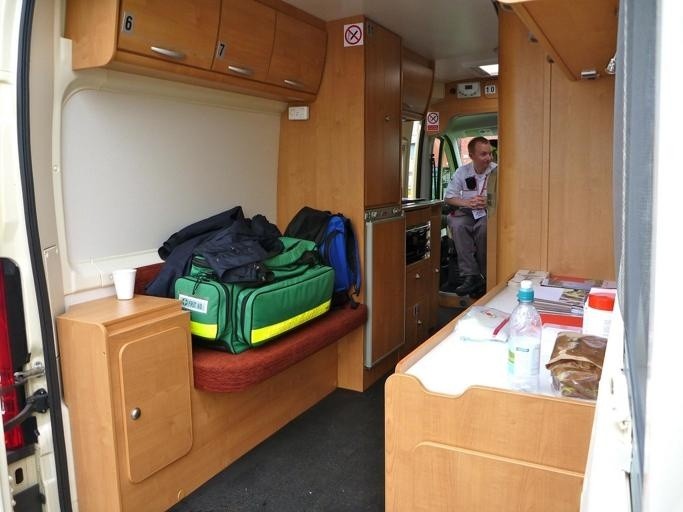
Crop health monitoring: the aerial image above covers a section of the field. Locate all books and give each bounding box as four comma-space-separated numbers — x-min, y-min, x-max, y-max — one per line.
505, 268, 608, 308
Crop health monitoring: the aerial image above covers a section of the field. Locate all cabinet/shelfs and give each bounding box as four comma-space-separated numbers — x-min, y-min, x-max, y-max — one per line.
54, 294, 196, 512
277, 15, 404, 210
397, 43, 435, 121
64, 0, 327, 106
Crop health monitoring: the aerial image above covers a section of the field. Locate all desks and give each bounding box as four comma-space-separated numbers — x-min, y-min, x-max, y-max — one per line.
384, 270, 613, 512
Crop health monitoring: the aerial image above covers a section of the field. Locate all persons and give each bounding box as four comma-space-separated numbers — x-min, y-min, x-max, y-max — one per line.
444, 136, 498, 302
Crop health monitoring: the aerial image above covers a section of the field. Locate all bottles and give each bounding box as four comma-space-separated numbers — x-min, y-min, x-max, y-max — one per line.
506, 280, 543, 395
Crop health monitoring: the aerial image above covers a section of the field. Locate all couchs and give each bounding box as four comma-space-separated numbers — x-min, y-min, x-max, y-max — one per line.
134, 257, 369, 394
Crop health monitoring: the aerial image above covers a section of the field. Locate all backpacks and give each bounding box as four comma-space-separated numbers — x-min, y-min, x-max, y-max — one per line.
284, 207, 362, 308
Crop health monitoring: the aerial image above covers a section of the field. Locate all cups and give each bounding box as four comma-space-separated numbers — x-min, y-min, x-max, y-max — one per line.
111, 266, 138, 300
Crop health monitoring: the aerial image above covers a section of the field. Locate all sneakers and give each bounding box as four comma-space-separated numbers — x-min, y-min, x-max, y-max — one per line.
456, 276, 486, 300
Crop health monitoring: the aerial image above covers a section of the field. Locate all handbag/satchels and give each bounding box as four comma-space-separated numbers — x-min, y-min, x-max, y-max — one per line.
175, 235, 336, 355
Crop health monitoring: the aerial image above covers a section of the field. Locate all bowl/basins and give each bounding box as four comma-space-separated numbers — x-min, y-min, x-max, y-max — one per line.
589, 293, 614, 310
